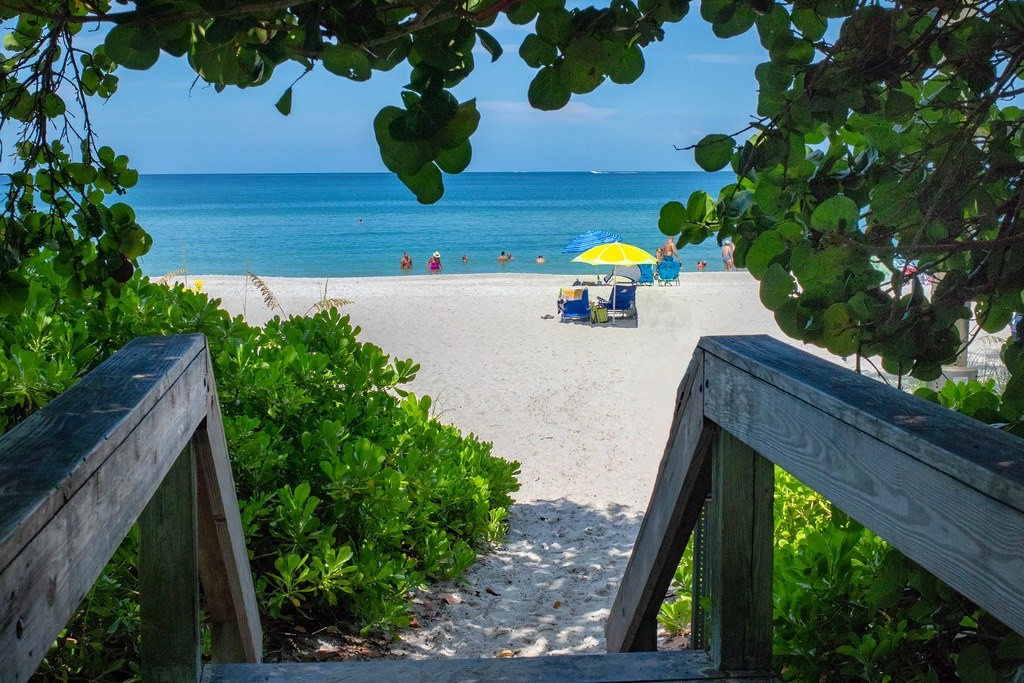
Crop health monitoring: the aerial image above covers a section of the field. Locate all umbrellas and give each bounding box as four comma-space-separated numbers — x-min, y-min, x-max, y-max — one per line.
561, 229, 624, 281
571, 241, 659, 322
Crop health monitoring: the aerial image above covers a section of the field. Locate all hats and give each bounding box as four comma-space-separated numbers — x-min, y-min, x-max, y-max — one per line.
433, 251, 440, 258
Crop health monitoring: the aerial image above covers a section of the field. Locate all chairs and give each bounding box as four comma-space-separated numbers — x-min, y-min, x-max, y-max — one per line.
597, 284, 639, 328
637, 269, 655, 287
656, 261, 683, 286
557, 286, 596, 324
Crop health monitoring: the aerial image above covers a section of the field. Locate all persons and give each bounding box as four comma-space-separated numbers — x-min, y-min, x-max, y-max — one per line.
401, 236, 743, 271
1008, 313, 1022, 336
